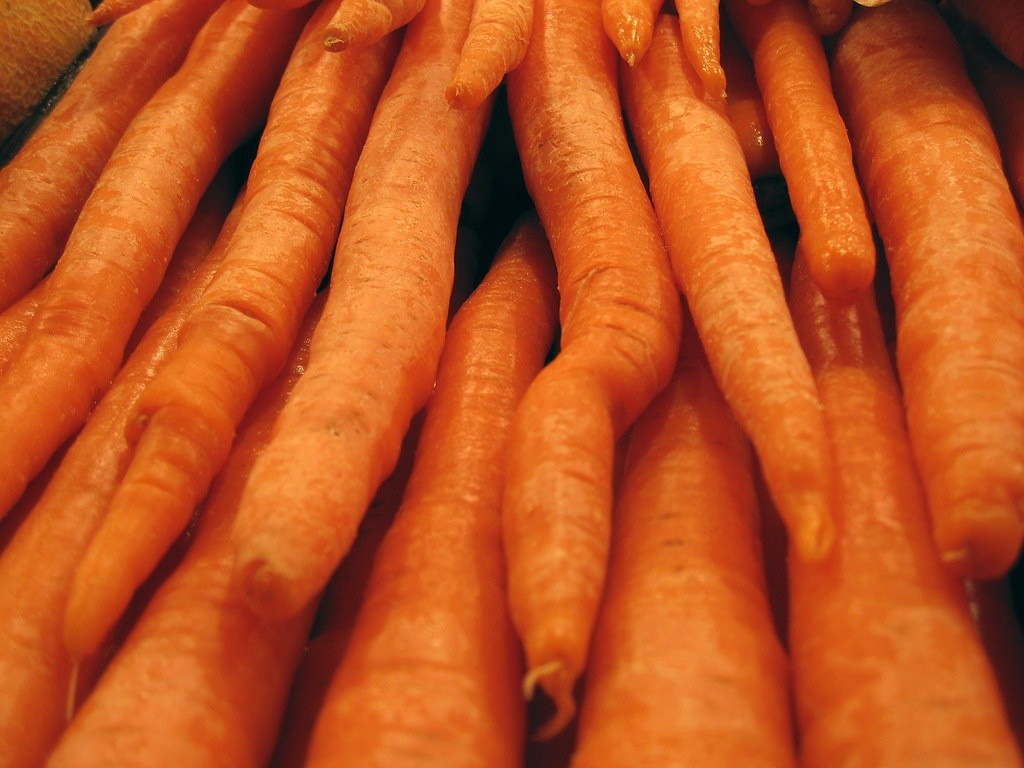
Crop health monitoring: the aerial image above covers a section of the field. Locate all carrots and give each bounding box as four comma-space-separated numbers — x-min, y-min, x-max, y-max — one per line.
0, 0, 1024, 768
